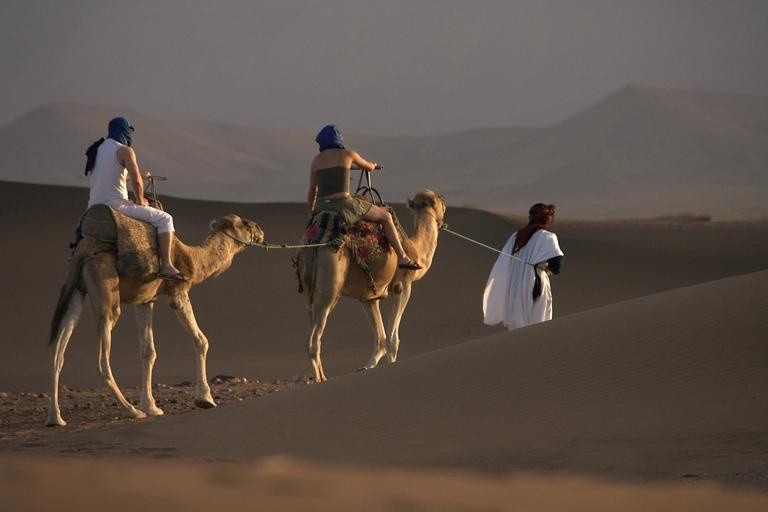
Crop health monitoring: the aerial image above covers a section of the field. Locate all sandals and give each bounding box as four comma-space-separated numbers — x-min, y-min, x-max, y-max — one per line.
157, 269, 189, 282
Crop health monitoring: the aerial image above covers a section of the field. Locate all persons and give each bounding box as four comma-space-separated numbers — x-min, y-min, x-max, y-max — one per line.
304, 122, 424, 271
66, 113, 190, 282
479, 201, 564, 332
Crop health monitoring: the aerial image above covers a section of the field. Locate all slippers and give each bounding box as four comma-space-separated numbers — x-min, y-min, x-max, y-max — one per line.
399, 259, 423, 270
